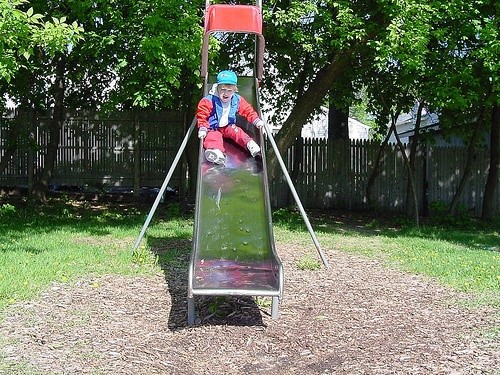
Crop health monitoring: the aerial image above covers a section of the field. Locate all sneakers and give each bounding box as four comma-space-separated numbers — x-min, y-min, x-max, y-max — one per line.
205, 148, 226, 165
248, 140, 261, 158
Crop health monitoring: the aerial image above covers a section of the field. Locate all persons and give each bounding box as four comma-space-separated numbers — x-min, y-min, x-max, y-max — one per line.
193, 70, 265, 166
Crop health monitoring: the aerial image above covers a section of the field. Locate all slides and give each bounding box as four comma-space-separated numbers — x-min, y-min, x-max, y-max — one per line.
187, 72, 283, 322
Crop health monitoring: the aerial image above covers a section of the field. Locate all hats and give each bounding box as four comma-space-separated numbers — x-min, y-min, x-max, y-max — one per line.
216, 70, 238, 85
